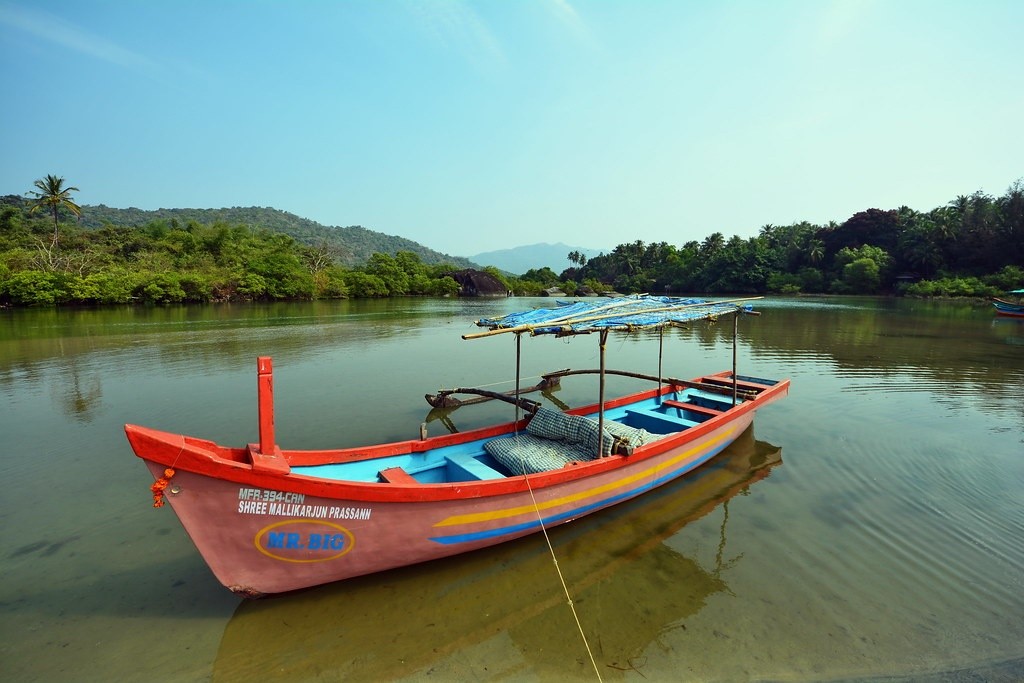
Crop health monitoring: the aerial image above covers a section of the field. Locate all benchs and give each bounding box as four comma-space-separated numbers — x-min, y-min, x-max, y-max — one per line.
625, 407, 700, 428
444, 451, 508, 481
380, 466, 420, 483
688, 392, 745, 409
663, 400, 725, 416
703, 377, 771, 393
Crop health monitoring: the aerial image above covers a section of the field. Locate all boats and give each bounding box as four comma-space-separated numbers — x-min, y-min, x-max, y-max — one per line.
993, 289, 1024, 317
208, 422, 787, 682
124, 293, 791, 602
